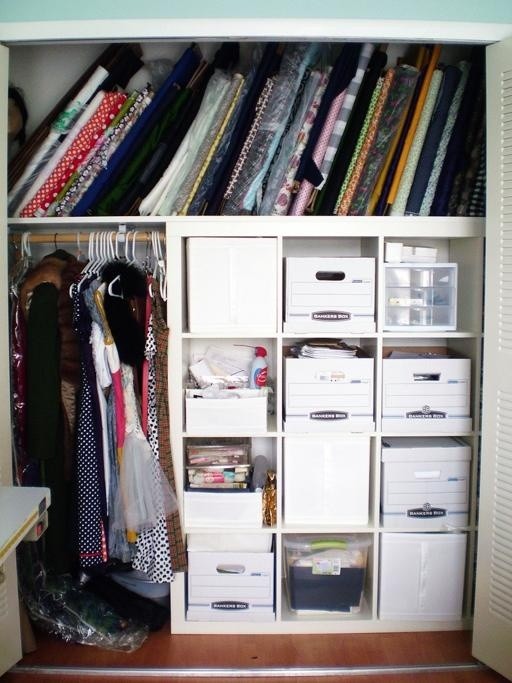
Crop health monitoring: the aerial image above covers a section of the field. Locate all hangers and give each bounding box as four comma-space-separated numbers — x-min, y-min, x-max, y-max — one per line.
6, 231, 167, 304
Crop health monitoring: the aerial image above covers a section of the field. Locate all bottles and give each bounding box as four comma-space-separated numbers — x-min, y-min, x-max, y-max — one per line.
249, 347, 267, 390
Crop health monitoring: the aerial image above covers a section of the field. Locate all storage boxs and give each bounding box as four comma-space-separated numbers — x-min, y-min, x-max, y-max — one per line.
183, 531, 279, 625
281, 535, 372, 616
184, 236, 279, 333
377, 531, 469, 620
380, 258, 458, 331
183, 381, 274, 436
284, 254, 379, 336
379, 344, 471, 433
280, 342, 378, 436
381, 436, 470, 530
181, 438, 276, 530
285, 437, 378, 530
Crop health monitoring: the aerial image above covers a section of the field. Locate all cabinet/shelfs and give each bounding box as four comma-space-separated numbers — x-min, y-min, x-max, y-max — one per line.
0, 17, 512, 682
